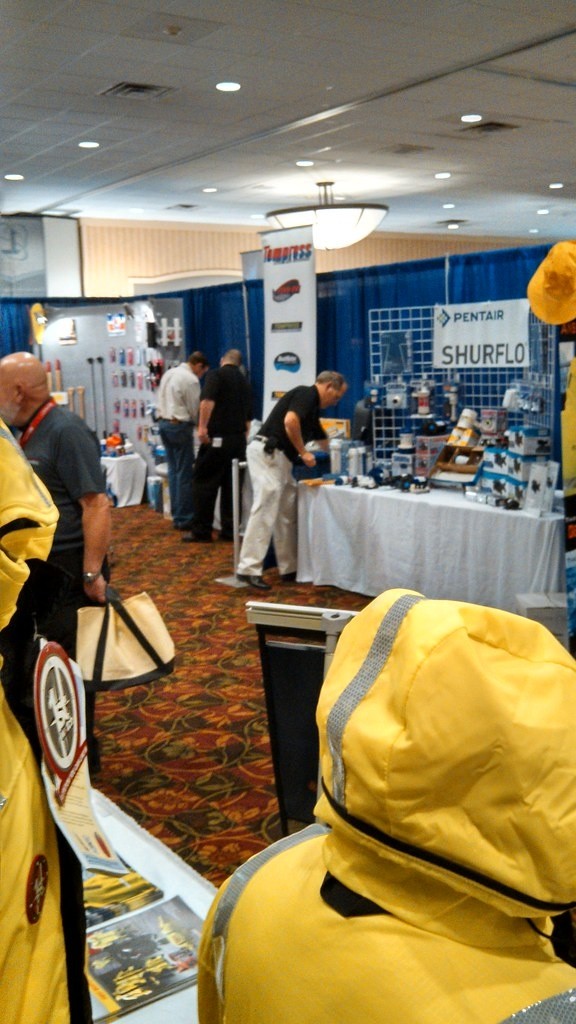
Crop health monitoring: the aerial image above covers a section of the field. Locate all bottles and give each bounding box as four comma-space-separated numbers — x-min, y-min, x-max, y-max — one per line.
327, 439, 366, 476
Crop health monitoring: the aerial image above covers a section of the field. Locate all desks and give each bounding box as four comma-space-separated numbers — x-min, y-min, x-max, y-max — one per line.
101, 453, 147, 507
294, 477, 566, 615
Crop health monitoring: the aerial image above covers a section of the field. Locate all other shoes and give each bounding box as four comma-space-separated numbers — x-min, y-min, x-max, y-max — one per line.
219, 530, 234, 542
181, 533, 212, 542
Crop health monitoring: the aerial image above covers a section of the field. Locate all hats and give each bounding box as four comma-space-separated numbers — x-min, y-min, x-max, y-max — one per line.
527, 240, 576, 325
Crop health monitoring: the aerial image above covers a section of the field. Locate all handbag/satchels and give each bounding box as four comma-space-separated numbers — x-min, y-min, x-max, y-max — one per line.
75, 585, 176, 691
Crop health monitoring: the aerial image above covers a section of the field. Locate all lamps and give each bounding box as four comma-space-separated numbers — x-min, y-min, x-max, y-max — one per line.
264, 181, 388, 253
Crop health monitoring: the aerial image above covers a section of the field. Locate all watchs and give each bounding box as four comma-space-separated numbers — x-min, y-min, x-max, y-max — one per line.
83, 573, 101, 581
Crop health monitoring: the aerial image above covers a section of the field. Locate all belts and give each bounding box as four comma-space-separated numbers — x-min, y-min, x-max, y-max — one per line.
254, 437, 267, 444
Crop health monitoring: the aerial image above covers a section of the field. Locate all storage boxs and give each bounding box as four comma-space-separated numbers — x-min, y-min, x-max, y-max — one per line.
391, 409, 560, 517
515, 591, 570, 653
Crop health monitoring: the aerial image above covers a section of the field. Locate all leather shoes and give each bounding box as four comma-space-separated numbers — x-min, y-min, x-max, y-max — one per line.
236, 572, 272, 591
281, 572, 298, 581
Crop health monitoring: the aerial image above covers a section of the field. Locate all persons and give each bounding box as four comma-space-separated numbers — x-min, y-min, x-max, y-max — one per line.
0, 350, 111, 1024
158, 349, 254, 542
199, 587, 576, 1024
236, 371, 348, 589
527, 241, 576, 552
